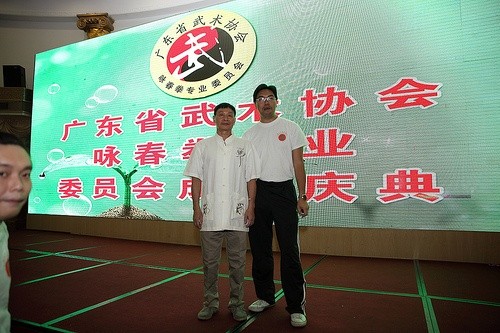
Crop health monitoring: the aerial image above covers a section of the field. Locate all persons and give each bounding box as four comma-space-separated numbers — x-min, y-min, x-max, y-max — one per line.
0, 131, 32, 333
242, 84, 309, 326
184, 103, 260, 321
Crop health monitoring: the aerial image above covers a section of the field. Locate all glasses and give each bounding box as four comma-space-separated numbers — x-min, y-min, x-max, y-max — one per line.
256, 95, 277, 102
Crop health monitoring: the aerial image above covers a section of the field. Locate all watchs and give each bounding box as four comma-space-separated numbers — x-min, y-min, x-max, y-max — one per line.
298, 195, 306, 200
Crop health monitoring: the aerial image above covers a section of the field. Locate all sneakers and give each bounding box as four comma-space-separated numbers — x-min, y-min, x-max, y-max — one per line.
291, 313, 307, 327
230, 308, 248, 321
198, 307, 219, 319
248, 300, 273, 312
